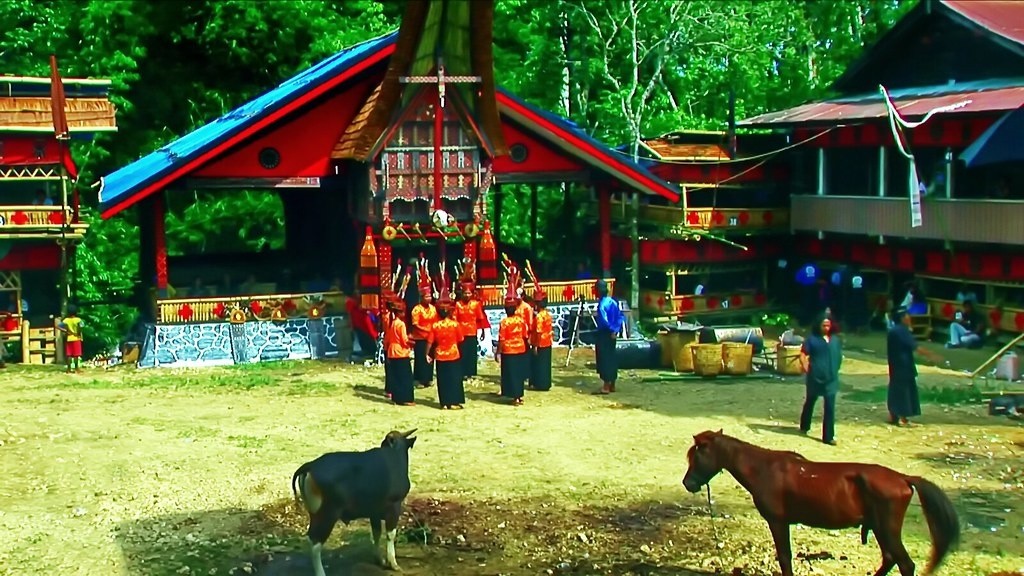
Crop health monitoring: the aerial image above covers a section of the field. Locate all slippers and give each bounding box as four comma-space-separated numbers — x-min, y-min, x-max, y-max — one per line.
592, 390, 609, 395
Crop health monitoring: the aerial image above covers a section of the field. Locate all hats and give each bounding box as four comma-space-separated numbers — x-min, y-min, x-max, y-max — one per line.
501, 252, 523, 307
890, 306, 907, 316
454, 257, 477, 290
595, 280, 607, 296
415, 252, 432, 296
382, 258, 412, 310
433, 260, 456, 310
524, 259, 547, 301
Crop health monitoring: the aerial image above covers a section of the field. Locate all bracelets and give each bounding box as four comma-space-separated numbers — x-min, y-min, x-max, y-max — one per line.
64, 329, 67, 332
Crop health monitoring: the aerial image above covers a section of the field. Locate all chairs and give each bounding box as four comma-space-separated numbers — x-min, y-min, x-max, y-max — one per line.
903, 301, 933, 332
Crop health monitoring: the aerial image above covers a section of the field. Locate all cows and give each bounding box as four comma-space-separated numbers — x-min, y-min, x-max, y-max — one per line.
291, 429, 417, 576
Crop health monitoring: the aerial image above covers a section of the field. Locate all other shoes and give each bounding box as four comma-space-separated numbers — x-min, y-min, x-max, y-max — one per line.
947, 343, 960, 348
386, 393, 391, 398
886, 417, 918, 427
404, 402, 417, 406
443, 404, 463, 410
512, 400, 524, 406
822, 439, 836, 445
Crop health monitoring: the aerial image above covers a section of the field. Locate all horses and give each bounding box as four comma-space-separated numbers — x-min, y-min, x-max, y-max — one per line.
682, 430, 959, 576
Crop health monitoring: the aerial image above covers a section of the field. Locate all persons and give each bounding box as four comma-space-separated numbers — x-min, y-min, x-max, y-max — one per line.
799, 314, 842, 446
55, 304, 84, 374
591, 279, 625, 394
381, 282, 491, 409
888, 305, 922, 427
948, 299, 986, 348
495, 271, 554, 406
